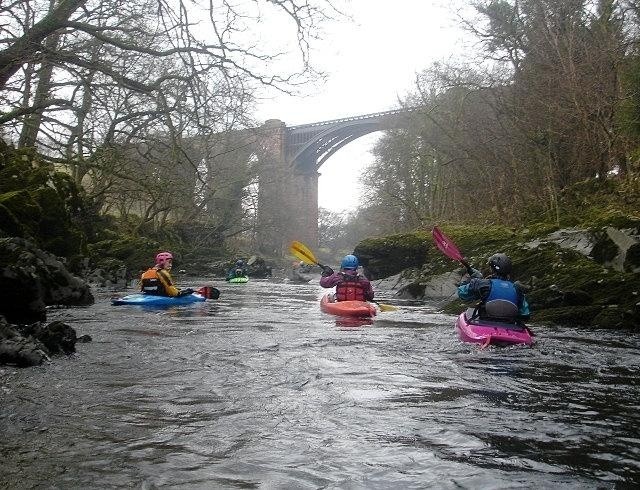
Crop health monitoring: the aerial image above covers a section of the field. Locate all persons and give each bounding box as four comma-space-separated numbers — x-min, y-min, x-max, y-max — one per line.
140, 251, 194, 298
458, 253, 530, 325
225, 260, 245, 281
297, 261, 305, 274
320, 255, 374, 302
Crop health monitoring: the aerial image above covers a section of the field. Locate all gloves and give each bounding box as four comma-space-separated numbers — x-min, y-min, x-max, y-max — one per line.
186, 288, 193, 294
466, 268, 483, 281
321, 265, 334, 277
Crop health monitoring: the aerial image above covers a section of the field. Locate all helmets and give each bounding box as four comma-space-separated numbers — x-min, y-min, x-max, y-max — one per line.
155, 252, 173, 264
235, 260, 243, 268
486, 252, 512, 277
341, 254, 359, 270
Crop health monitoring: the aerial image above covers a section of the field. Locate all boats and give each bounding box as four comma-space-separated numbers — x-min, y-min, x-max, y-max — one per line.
117, 292, 205, 305
322, 294, 381, 315
458, 311, 532, 344
231, 276, 248, 282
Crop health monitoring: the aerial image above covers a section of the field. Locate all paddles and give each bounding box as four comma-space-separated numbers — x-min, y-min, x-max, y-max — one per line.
193, 287, 219, 300
432, 226, 473, 273
289, 241, 397, 311
